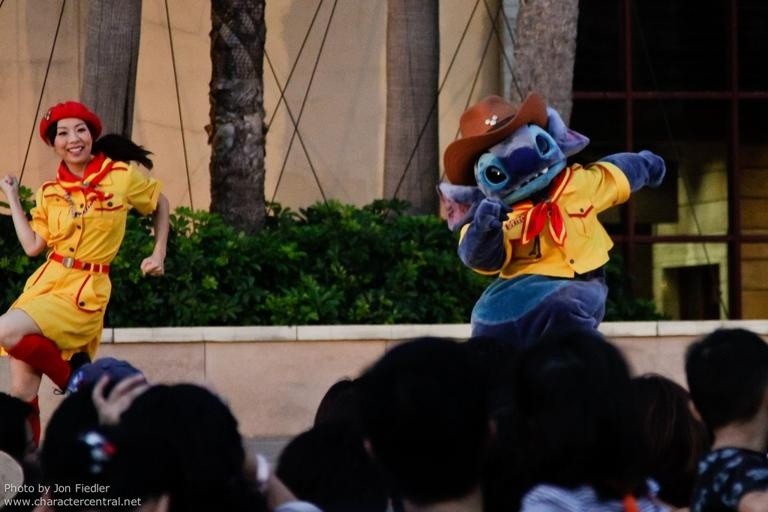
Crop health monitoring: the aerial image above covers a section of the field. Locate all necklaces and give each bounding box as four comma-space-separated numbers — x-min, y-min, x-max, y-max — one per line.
63, 190, 97, 218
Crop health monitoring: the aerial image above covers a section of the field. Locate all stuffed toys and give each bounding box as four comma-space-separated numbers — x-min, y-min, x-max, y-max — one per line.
432, 90, 667, 358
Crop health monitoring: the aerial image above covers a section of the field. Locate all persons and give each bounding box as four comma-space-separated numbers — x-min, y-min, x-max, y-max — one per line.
0, 100, 171, 451
0, 312, 768, 510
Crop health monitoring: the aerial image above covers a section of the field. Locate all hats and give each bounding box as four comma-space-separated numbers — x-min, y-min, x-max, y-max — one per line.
444, 92, 547, 185
40, 102, 102, 146
